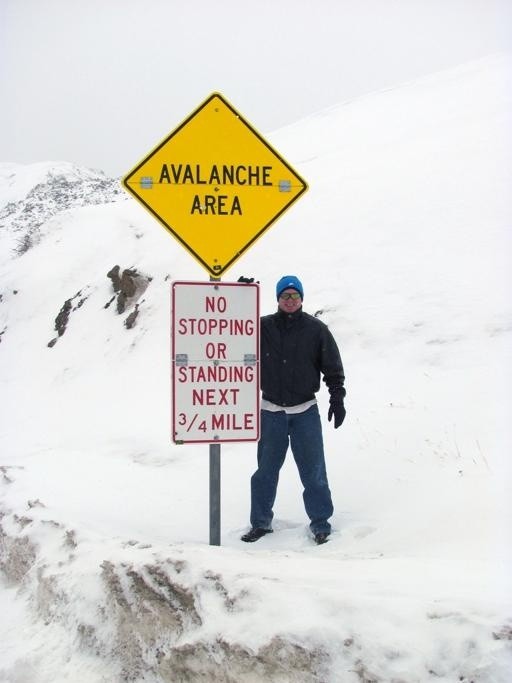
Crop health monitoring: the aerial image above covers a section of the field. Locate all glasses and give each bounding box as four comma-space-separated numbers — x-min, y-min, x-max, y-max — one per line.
278, 290, 302, 300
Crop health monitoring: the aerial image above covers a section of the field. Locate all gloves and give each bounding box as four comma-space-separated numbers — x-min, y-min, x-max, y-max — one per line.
327, 392, 348, 428
238, 274, 260, 285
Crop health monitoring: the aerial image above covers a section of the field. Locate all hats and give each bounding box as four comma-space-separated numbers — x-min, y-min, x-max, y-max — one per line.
275, 274, 304, 301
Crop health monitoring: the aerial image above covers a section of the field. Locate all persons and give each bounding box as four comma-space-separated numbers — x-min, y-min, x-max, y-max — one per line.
237, 275, 345, 548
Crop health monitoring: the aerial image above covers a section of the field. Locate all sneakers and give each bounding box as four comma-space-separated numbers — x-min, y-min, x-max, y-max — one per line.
314, 529, 331, 543
240, 525, 274, 543
